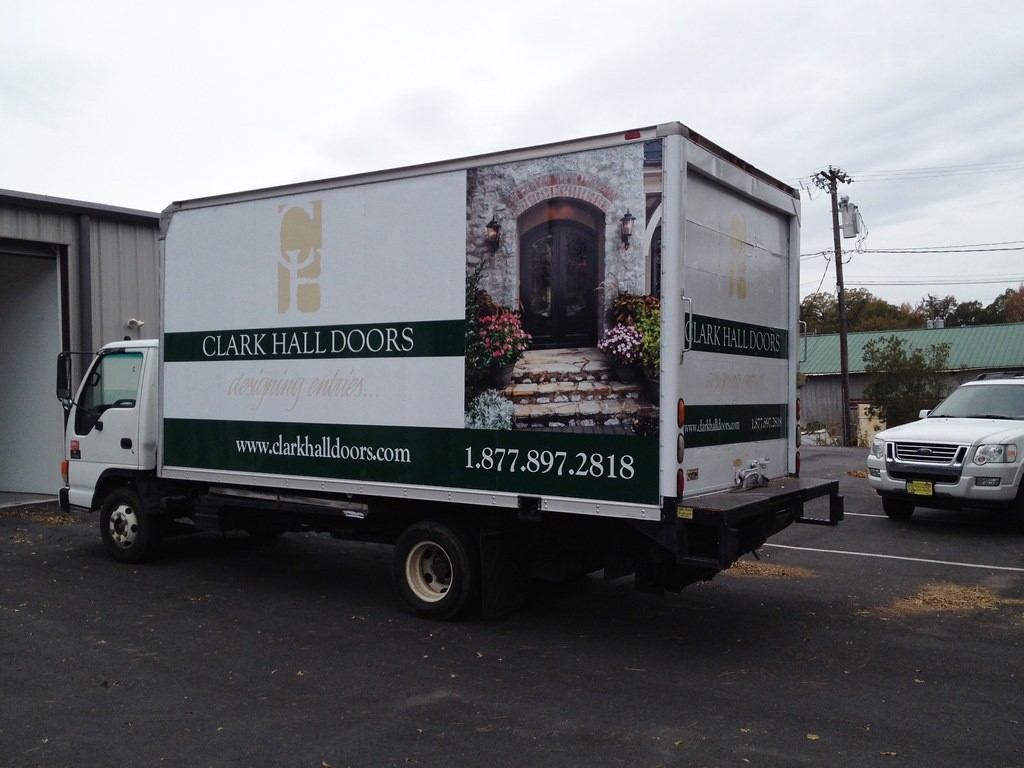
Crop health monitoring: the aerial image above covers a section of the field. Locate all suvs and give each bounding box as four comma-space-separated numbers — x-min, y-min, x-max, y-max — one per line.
865, 365, 1023, 525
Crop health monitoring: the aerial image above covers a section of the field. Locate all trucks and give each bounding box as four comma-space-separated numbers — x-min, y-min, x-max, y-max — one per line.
52, 118, 847, 625
